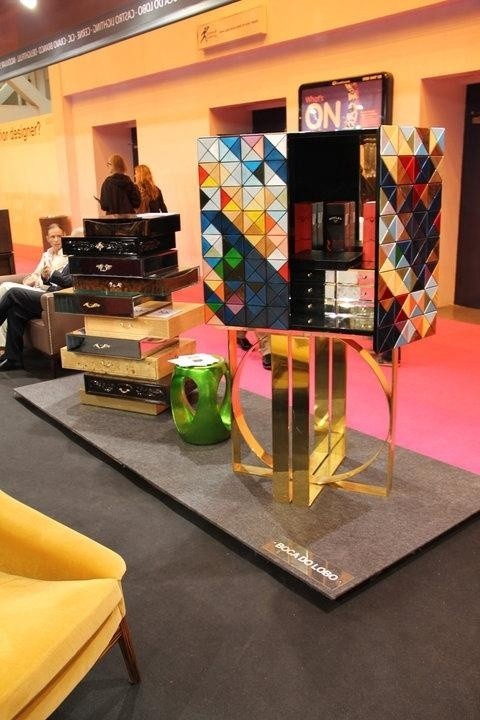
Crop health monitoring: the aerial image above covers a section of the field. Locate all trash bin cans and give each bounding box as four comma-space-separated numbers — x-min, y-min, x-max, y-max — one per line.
39, 216, 72, 253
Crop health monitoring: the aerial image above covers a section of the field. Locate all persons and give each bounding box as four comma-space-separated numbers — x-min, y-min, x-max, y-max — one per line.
0, 223, 70, 358
133, 165, 169, 214
99, 154, 142, 215
0, 227, 86, 371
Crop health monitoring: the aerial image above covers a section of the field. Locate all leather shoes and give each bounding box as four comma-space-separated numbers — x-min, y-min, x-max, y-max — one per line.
0, 358, 24, 370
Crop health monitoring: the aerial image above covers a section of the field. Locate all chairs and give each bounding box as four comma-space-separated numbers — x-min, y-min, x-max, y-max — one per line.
1, 491, 141, 719
0, 272, 86, 369
